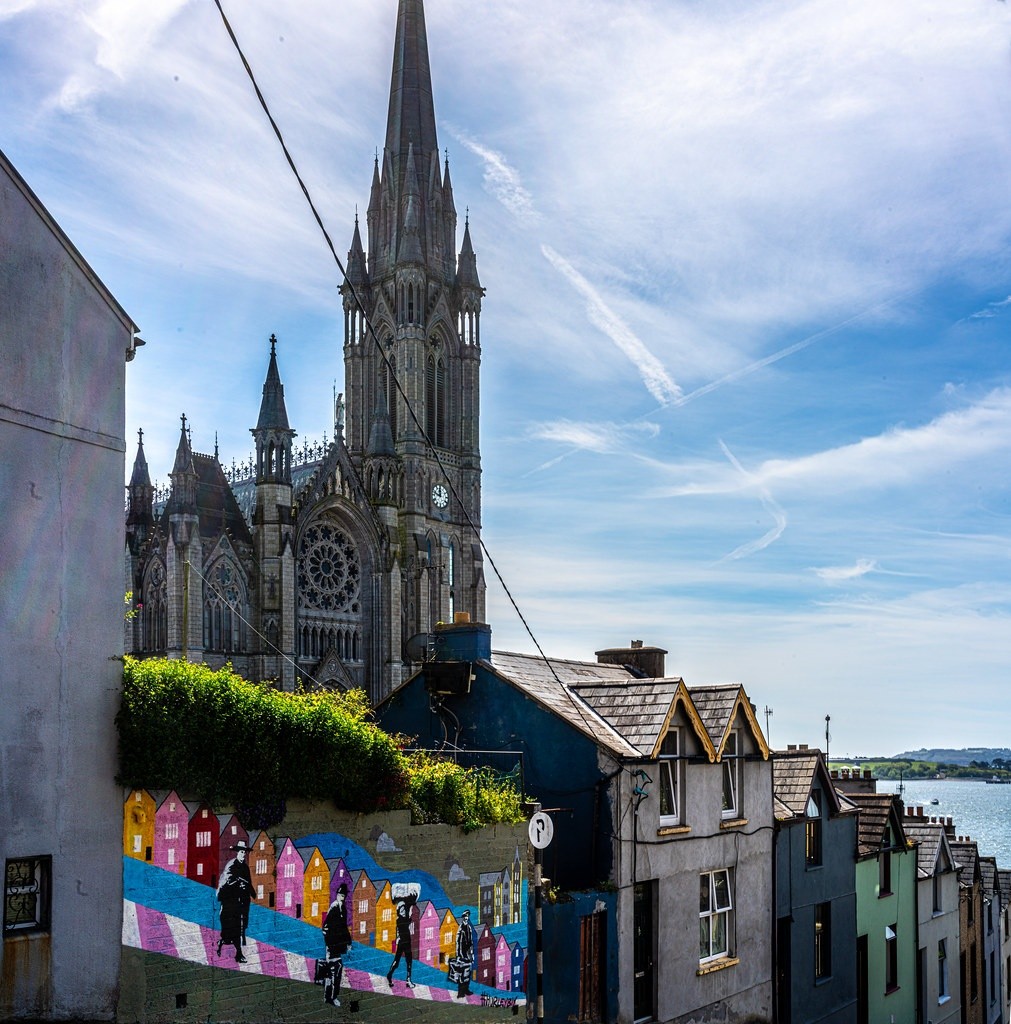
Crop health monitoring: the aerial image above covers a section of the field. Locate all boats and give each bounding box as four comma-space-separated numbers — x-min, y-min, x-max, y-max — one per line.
931, 774, 945, 780
931, 798, 939, 805
985, 776, 1011, 784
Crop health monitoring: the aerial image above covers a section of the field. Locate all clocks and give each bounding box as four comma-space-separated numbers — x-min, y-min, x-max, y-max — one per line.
431, 483, 450, 510
